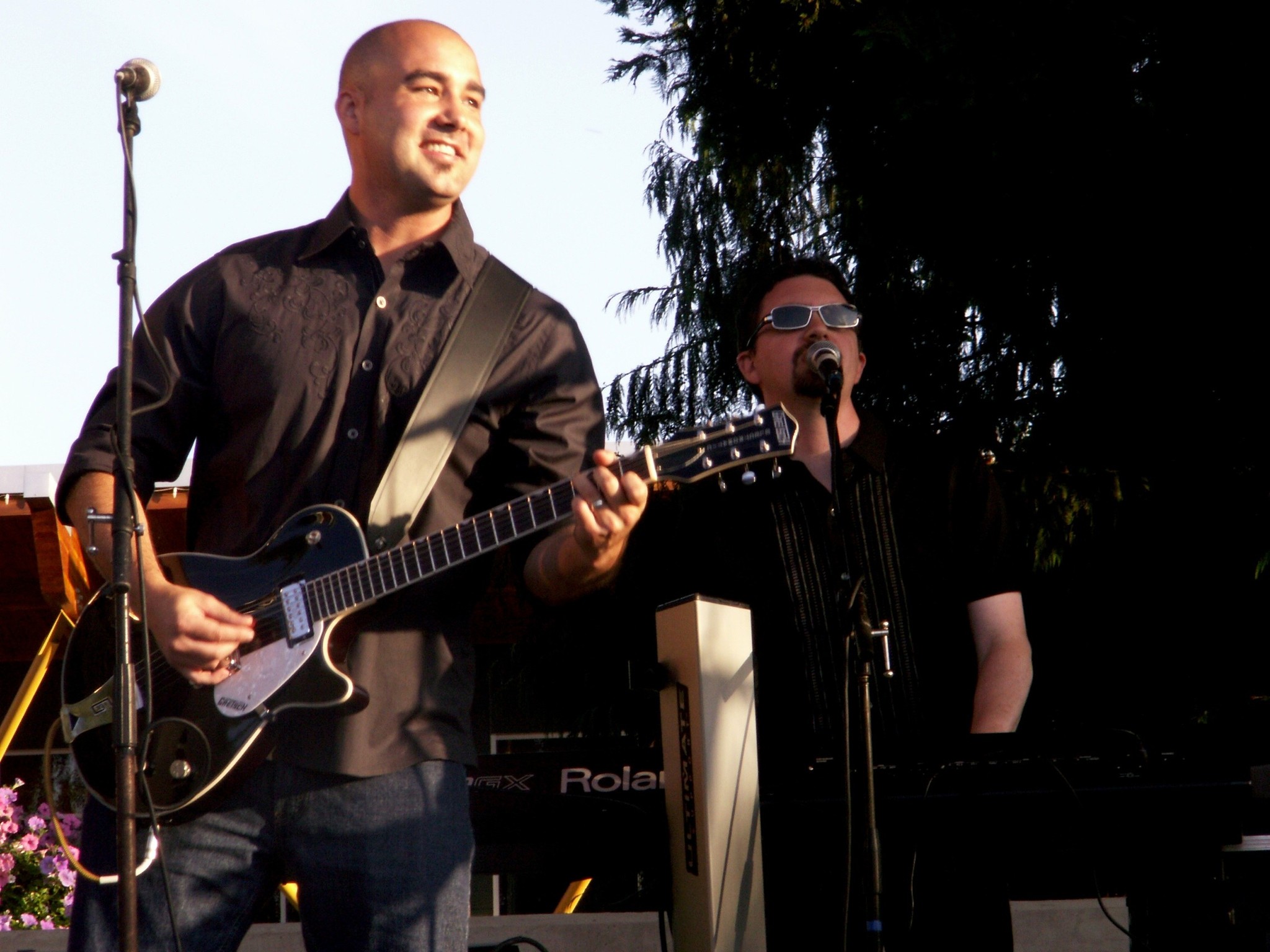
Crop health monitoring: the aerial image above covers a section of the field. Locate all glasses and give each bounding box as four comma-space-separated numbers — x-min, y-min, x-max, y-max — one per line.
744, 303, 863, 349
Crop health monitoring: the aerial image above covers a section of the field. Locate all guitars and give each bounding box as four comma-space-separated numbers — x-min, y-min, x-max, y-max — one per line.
63, 402, 797, 816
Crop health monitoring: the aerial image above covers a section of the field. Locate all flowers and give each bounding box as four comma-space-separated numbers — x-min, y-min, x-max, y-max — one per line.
0, 776, 82, 935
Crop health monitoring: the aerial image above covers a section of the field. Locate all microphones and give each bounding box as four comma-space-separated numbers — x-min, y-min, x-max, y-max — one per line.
114, 58, 161, 102
806, 341, 844, 391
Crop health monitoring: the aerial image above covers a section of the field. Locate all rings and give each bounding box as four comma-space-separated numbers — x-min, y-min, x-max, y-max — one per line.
588, 498, 605, 512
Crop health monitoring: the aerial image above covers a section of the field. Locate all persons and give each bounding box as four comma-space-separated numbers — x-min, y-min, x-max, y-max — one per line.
679, 254, 1032, 952
53, 19, 649, 952
